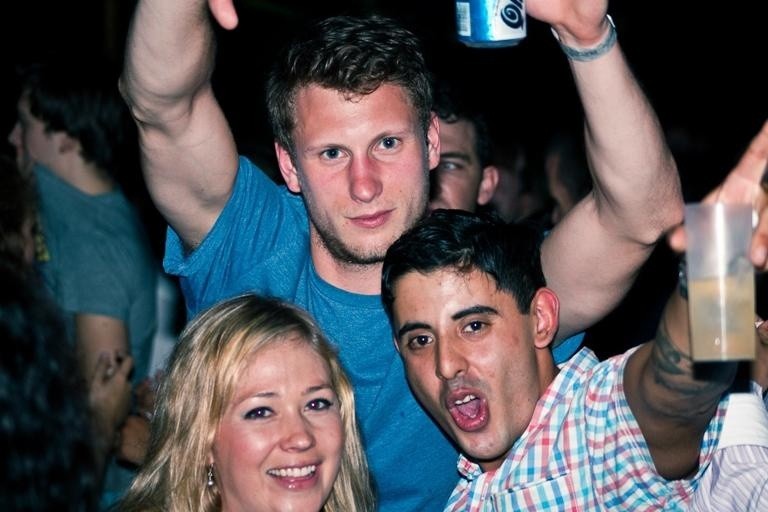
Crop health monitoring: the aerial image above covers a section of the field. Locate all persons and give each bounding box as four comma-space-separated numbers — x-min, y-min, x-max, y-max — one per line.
1, 0, 768, 512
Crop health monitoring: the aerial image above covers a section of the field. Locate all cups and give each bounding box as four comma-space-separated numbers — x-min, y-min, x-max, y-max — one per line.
685, 203, 756, 360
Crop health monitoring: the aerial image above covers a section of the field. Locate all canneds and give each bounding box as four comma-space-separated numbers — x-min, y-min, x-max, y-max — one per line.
456, 0, 527, 48
